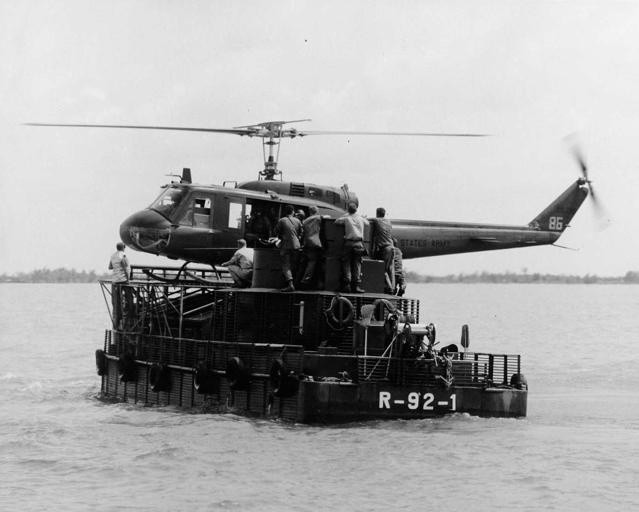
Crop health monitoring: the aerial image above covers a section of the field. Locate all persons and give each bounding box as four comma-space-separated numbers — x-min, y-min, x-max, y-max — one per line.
108, 242, 133, 285
274, 204, 407, 296
222, 239, 255, 287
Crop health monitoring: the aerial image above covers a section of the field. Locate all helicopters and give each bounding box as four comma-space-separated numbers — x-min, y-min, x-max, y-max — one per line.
17, 115, 605, 269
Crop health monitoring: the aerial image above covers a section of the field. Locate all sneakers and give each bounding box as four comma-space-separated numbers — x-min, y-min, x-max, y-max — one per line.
231, 281, 244, 288
353, 287, 365, 293
280, 285, 295, 292
336, 285, 352, 293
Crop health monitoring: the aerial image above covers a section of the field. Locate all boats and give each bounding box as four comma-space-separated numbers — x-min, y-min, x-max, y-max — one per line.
78, 256, 530, 429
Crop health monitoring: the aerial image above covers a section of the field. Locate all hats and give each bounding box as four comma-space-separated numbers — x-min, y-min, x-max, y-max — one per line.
237, 239, 246, 245
